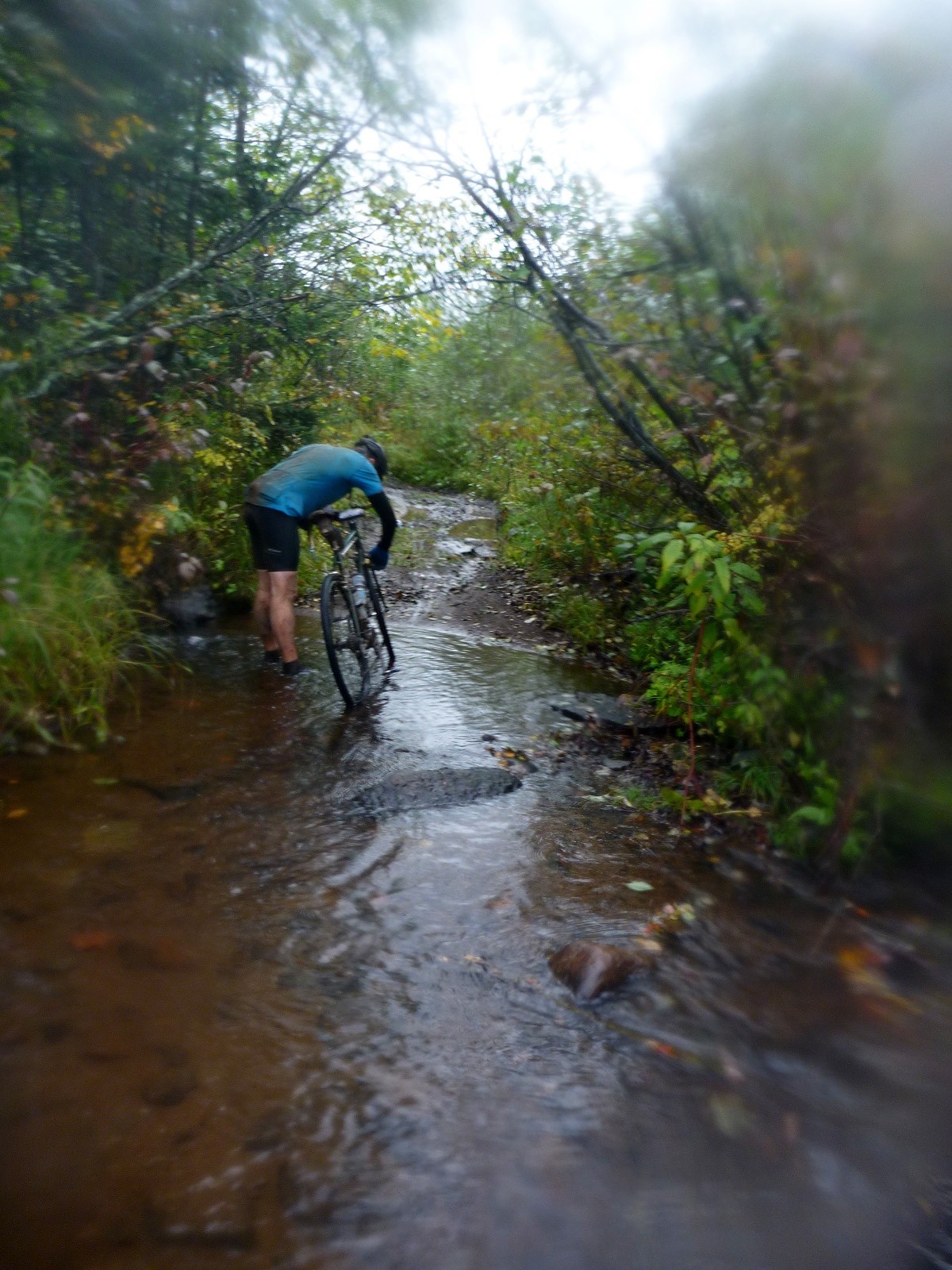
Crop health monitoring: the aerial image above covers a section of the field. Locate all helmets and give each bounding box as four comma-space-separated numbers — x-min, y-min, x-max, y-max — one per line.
355, 436, 386, 481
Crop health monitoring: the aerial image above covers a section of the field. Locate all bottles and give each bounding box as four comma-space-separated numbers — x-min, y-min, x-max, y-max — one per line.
351, 573, 366, 606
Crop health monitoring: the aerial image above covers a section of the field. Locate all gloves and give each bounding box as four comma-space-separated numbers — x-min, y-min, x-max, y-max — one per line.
366, 544, 387, 570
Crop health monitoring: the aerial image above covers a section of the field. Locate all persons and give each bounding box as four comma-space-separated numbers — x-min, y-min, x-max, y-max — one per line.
242, 438, 397, 675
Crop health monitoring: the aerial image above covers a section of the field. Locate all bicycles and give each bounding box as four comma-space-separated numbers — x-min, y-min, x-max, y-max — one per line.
304, 501, 404, 711
